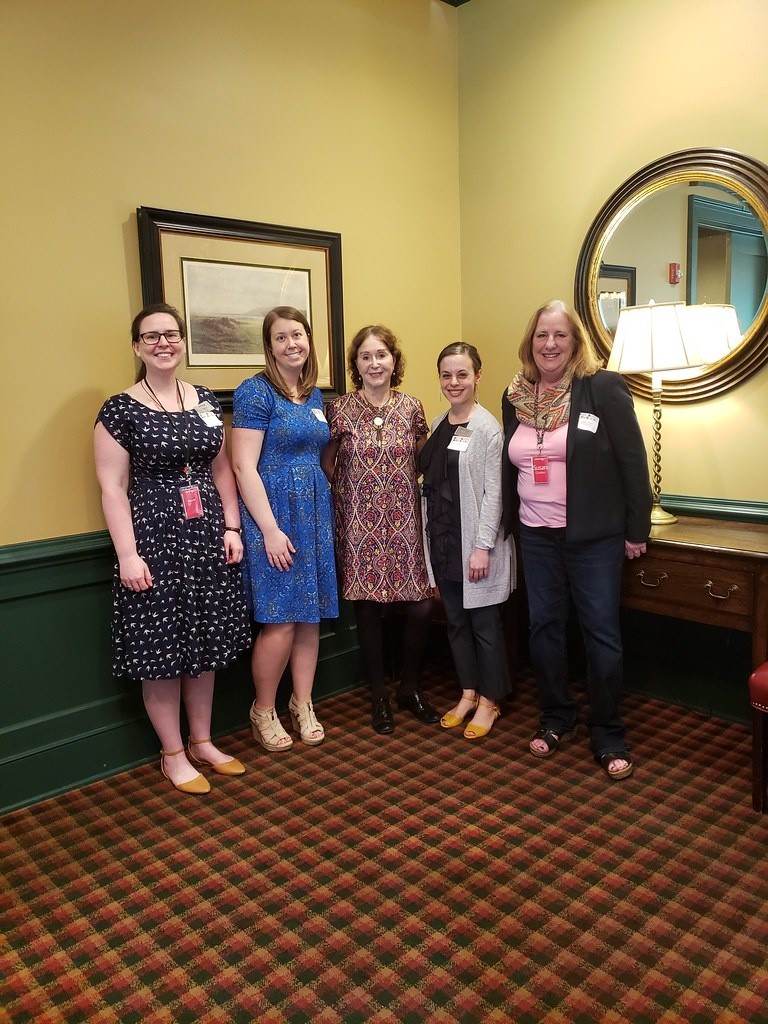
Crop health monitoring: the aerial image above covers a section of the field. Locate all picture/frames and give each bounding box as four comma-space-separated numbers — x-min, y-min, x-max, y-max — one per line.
596, 265, 637, 328
128, 206, 341, 412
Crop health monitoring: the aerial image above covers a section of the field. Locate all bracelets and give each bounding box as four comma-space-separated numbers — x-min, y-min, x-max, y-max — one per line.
225, 526, 243, 535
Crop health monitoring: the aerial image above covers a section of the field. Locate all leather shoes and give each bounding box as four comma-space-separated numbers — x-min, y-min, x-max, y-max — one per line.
371, 694, 395, 734
397, 687, 441, 723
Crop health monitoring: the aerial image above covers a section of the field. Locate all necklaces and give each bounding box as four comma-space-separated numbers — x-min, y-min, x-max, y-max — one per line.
363, 386, 391, 449
139, 375, 186, 413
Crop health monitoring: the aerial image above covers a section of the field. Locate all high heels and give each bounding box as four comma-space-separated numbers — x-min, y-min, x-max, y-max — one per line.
249, 698, 294, 751
289, 693, 325, 746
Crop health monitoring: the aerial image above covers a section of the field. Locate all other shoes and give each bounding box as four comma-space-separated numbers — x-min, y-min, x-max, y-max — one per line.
159, 746, 210, 794
187, 735, 246, 775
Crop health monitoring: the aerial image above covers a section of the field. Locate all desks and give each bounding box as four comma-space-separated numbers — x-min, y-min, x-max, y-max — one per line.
620, 516, 768, 672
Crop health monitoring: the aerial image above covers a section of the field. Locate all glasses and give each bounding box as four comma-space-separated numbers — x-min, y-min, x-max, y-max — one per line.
140, 329, 185, 345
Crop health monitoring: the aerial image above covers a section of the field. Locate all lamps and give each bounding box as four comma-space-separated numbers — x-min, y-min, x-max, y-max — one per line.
593, 304, 703, 526
677, 302, 740, 369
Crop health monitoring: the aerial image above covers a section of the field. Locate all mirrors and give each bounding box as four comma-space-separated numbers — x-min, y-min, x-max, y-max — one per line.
576, 148, 768, 404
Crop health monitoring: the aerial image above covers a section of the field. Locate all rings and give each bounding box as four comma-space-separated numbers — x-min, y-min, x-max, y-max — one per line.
127, 586, 132, 589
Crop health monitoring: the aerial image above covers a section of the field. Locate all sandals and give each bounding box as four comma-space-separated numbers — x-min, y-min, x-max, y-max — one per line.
439, 693, 479, 728
528, 725, 578, 758
601, 751, 634, 779
463, 701, 501, 739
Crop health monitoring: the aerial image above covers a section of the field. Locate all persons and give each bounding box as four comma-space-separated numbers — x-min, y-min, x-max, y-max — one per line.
92, 304, 247, 794
416, 341, 519, 743
319, 326, 442, 734
231, 306, 335, 749
501, 300, 654, 778
